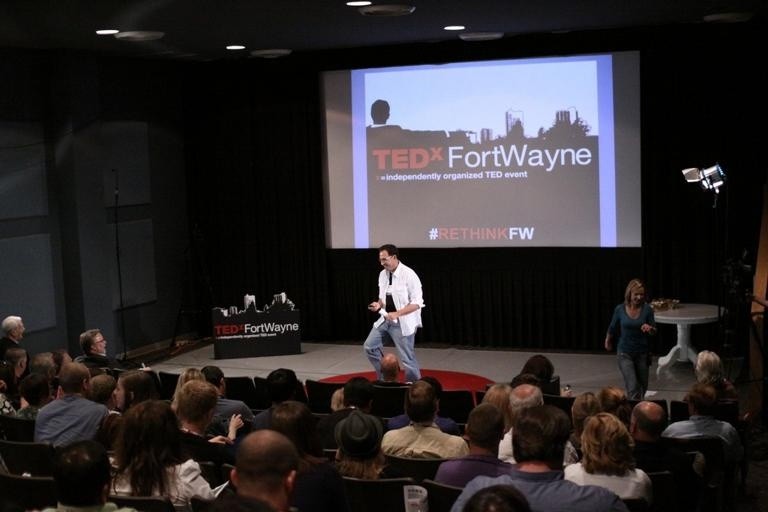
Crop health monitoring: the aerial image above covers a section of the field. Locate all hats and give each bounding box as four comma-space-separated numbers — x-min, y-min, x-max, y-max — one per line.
333, 410, 384, 462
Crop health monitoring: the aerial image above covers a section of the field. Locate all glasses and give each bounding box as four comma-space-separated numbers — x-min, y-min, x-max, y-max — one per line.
96, 338, 105, 343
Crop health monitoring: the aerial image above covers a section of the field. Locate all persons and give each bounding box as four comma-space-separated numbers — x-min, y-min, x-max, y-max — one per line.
497, 383, 545, 463
629, 402, 696, 472
110, 400, 230, 512
683, 350, 739, 401
35, 439, 139, 512
597, 385, 631, 428
386, 376, 461, 436
315, 376, 387, 459
563, 393, 601, 464
482, 354, 572, 404
253, 369, 298, 428
17, 373, 52, 420
461, 485, 532, 512
1, 315, 26, 360
209, 429, 300, 512
28, 352, 57, 384
177, 380, 243, 465
4, 347, 27, 385
361, 243, 426, 384
266, 402, 348, 511
34, 363, 111, 457
202, 366, 255, 425
449, 403, 630, 511
89, 375, 117, 413
113, 370, 162, 415
380, 381, 471, 460
563, 412, 653, 505
434, 402, 514, 489
334, 410, 408, 480
74, 328, 141, 380
660, 385, 746, 456
50, 349, 73, 397
604, 278, 657, 401
379, 351, 401, 381
170, 368, 204, 409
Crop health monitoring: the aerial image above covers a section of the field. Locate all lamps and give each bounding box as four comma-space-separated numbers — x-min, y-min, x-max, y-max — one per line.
682, 163, 728, 194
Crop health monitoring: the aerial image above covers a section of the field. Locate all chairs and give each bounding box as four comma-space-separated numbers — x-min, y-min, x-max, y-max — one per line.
302, 377, 358, 413
623, 396, 670, 435
253, 373, 307, 406
667, 437, 753, 501
0, 416, 38, 439
370, 384, 412, 418
436, 390, 476, 428
340, 472, 411, 508
0, 440, 59, 480
540, 392, 579, 420
222, 376, 260, 414
157, 371, 186, 401
380, 452, 444, 482
108, 491, 176, 512
668, 398, 741, 435
0, 481, 56, 510
114, 368, 159, 395
422, 479, 469, 512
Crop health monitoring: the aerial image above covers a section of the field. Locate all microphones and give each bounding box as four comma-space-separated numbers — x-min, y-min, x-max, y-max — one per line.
379, 308, 400, 325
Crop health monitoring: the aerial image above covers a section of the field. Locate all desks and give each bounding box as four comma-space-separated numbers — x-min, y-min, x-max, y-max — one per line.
647, 302, 729, 381
